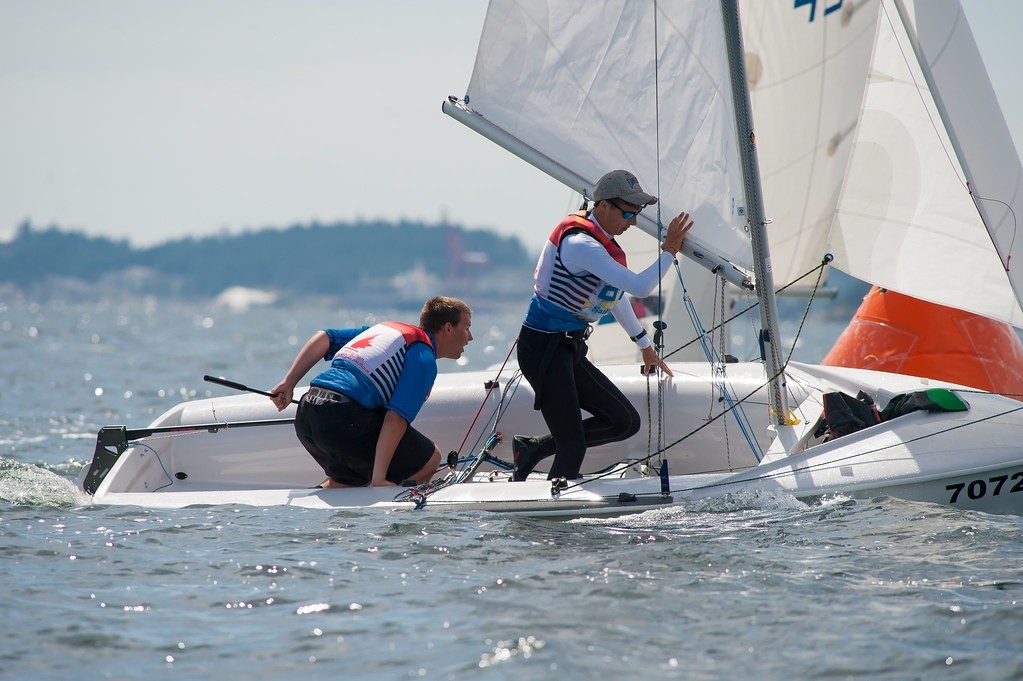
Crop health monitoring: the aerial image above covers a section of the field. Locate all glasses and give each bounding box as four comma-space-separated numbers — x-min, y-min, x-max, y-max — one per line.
610, 200, 642, 219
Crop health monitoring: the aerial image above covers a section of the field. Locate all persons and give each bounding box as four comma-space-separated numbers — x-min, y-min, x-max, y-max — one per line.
512, 170, 693, 482
270, 296, 474, 488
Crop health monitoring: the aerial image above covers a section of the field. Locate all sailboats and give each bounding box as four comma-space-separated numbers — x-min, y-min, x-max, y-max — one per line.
80, 0, 1022, 522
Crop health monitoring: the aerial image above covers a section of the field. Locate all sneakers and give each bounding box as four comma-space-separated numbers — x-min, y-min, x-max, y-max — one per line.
512, 435, 539, 481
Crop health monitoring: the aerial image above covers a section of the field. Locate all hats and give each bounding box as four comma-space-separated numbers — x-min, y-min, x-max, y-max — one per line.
593, 170, 657, 205
